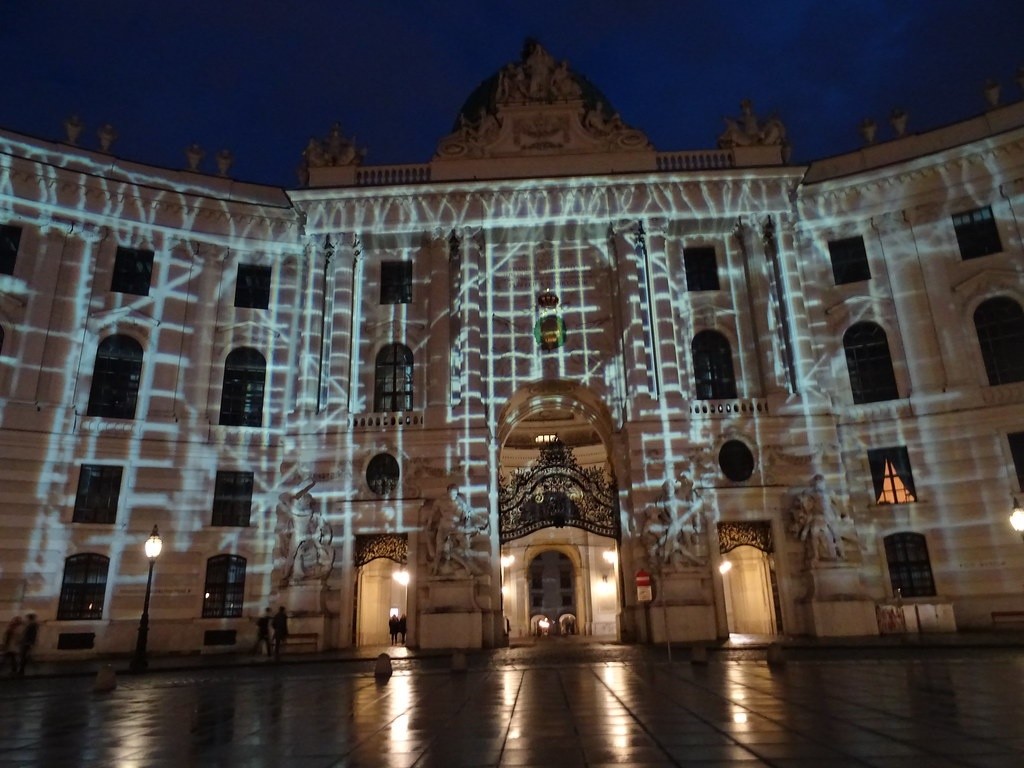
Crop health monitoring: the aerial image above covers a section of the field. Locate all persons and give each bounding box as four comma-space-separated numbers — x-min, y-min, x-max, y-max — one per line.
253, 607, 273, 658
505, 614, 511, 635
271, 604, 288, 659
388, 614, 400, 645
564, 617, 575, 635
399, 613, 408, 643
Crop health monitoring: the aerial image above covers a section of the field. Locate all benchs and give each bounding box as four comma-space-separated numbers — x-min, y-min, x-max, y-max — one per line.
991, 610, 1024, 632
271, 632, 319, 653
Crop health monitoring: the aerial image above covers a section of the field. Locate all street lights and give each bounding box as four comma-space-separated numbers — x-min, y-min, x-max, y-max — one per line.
128, 522, 163, 668
